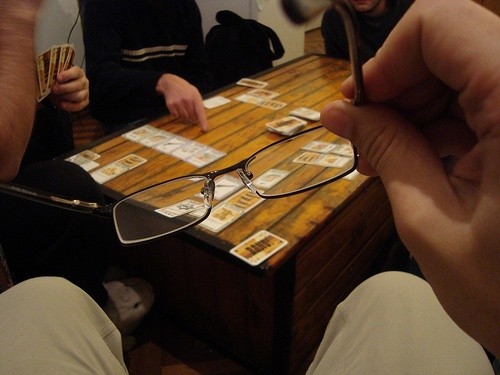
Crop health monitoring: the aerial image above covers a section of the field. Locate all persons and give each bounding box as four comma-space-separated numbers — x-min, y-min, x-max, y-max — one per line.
78, 0, 210, 134
321, 0, 414, 61
0, 0, 500, 375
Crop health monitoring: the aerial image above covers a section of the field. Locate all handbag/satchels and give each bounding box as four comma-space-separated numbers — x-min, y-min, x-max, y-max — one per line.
206, 9, 285, 79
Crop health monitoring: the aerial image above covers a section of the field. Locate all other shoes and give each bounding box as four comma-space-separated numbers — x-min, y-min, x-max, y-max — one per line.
98, 276, 155, 324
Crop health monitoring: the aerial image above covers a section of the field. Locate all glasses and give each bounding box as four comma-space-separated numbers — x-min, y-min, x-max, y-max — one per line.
0, 0, 363, 245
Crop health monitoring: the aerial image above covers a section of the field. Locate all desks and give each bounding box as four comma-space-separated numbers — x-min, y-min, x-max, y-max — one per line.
55, 53, 398, 375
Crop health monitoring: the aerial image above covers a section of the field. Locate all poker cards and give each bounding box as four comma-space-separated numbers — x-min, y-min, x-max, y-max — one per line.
65, 150, 103, 172
293, 139, 356, 168
121, 123, 225, 168
89, 153, 147, 185
154, 198, 205, 218
251, 167, 292, 189
265, 115, 306, 138
201, 95, 231, 110
291, 107, 321, 121
195, 174, 244, 201
31, 44, 75, 102
188, 187, 265, 233
236, 77, 267, 89
228, 229, 288, 266
235, 88, 288, 110
